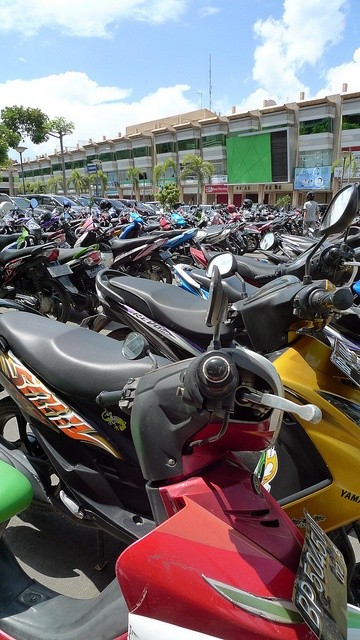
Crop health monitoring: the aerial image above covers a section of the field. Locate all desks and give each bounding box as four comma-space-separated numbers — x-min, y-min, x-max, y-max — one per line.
14, 146, 28, 194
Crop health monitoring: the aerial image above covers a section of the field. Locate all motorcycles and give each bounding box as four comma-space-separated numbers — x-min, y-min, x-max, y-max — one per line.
0, 202, 360, 344
0, 185, 360, 607
0, 265, 360, 640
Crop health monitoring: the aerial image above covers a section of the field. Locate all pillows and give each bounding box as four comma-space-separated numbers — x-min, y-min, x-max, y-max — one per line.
227, 204, 235, 212
63, 200, 71, 208
100, 200, 111, 209
243, 199, 252, 208
307, 193, 315, 201
41, 210, 52, 221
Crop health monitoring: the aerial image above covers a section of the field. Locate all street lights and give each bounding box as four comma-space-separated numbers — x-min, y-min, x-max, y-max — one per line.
14, 146, 28, 194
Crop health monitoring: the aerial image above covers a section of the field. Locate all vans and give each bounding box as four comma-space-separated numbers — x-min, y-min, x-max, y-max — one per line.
0, 192, 228, 216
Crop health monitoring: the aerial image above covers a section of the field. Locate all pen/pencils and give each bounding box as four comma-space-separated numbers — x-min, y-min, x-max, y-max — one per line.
0, 192, 228, 216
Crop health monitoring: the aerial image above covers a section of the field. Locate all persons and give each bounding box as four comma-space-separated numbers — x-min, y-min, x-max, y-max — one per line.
303, 192, 319, 239
